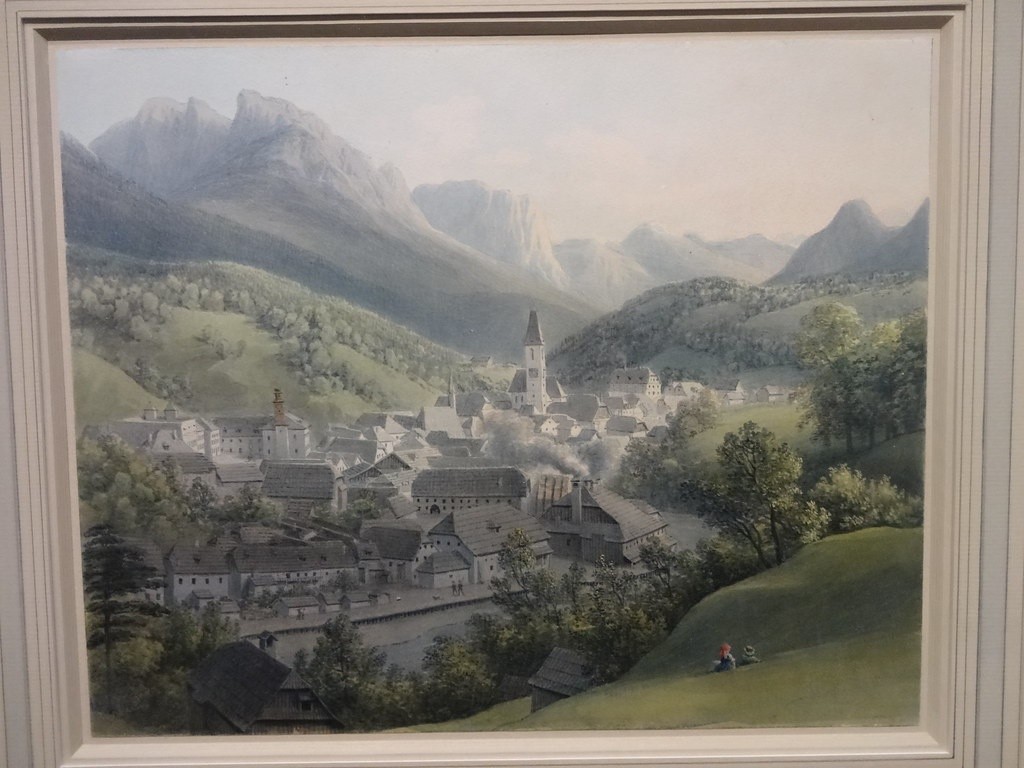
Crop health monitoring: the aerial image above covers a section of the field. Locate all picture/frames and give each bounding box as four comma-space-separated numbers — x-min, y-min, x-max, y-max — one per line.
0, 0, 1024, 768
